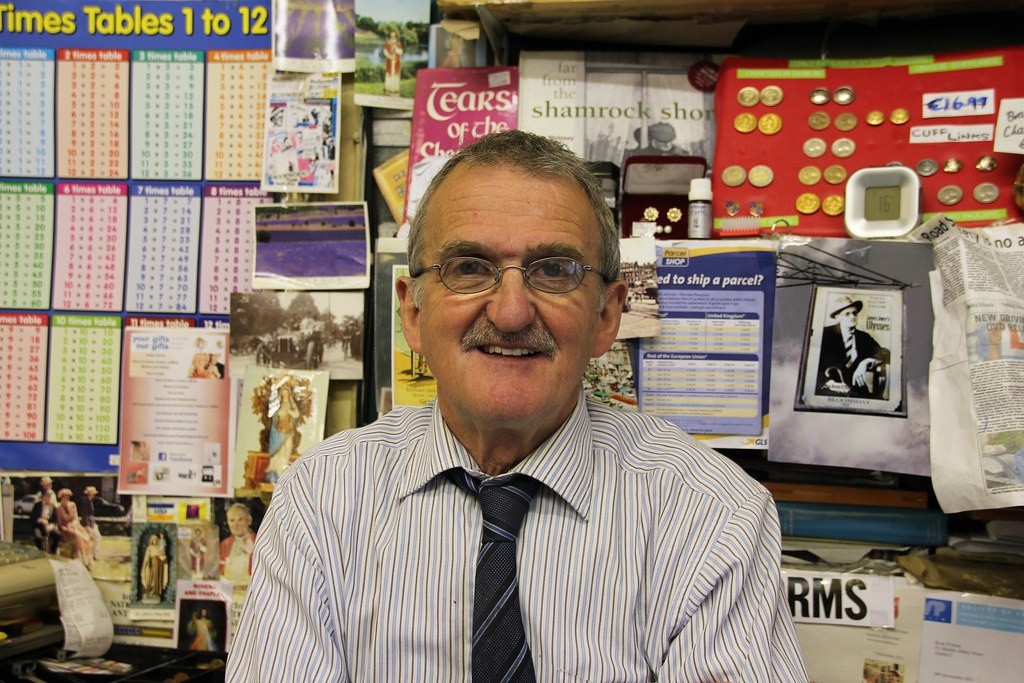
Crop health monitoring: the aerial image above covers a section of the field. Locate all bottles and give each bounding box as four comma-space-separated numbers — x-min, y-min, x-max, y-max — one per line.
687, 178, 713, 240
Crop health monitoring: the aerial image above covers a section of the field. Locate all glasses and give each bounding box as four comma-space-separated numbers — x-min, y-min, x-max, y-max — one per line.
413, 255, 616, 295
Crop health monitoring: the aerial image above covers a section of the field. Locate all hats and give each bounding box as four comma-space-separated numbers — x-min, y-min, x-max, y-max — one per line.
83, 486, 98, 495
40, 477, 53, 486
829, 297, 863, 319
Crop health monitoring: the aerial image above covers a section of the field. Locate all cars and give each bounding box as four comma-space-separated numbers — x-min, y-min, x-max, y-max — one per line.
92, 497, 126, 517
15, 494, 41, 517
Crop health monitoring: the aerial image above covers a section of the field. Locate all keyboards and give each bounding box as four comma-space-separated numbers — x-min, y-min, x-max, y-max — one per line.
0, 540, 71, 598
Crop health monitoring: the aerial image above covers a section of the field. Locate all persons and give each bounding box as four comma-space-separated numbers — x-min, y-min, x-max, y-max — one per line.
819, 294, 891, 400
29, 477, 102, 561
220, 134, 809, 683
220, 501, 257, 578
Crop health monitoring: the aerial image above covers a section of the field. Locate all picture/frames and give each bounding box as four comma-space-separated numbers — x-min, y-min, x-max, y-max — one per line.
793, 282, 909, 419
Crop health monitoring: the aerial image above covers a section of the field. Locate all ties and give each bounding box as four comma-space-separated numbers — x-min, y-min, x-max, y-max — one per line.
442, 467, 539, 683
845, 327, 855, 368
